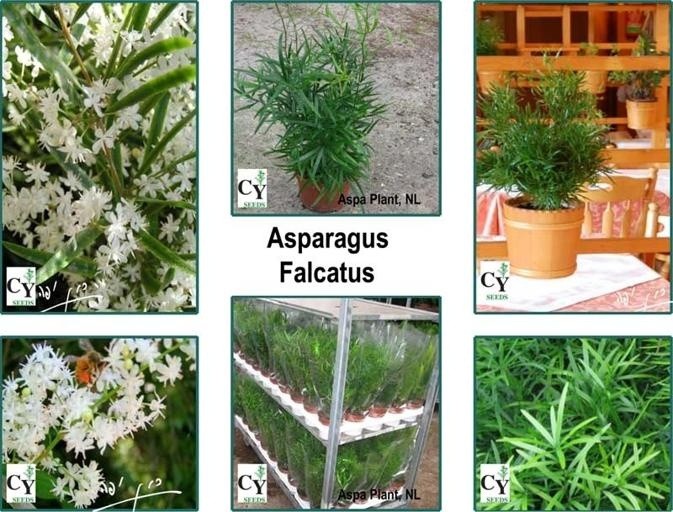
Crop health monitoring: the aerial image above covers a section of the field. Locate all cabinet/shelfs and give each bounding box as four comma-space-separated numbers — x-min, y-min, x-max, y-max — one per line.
230, 298, 439, 510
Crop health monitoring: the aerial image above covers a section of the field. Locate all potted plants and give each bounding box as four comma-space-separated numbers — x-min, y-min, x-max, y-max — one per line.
234, 300, 435, 505
235, 6, 392, 211
473, 15, 673, 279
